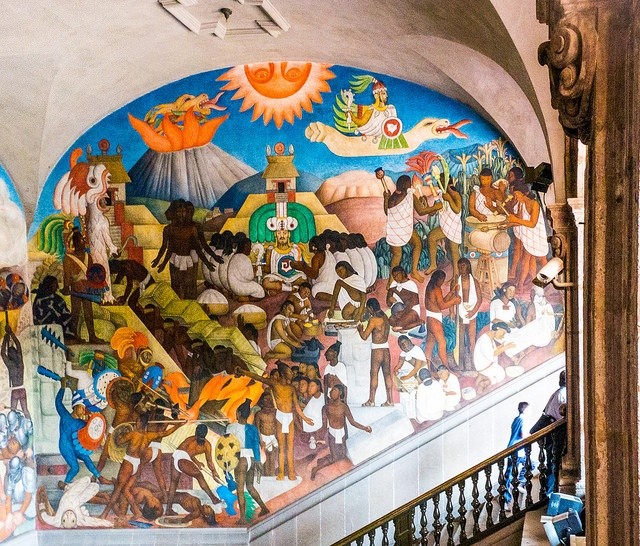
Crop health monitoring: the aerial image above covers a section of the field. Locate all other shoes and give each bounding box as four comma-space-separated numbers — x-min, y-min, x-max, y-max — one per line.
518, 486, 525, 494
504, 502, 511, 512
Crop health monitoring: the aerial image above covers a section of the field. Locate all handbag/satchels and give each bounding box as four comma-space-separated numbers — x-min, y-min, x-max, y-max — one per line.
529, 415, 557, 449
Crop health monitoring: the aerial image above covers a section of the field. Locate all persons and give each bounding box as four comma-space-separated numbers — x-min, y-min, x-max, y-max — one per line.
502, 402, 534, 511
530, 370, 567, 499
